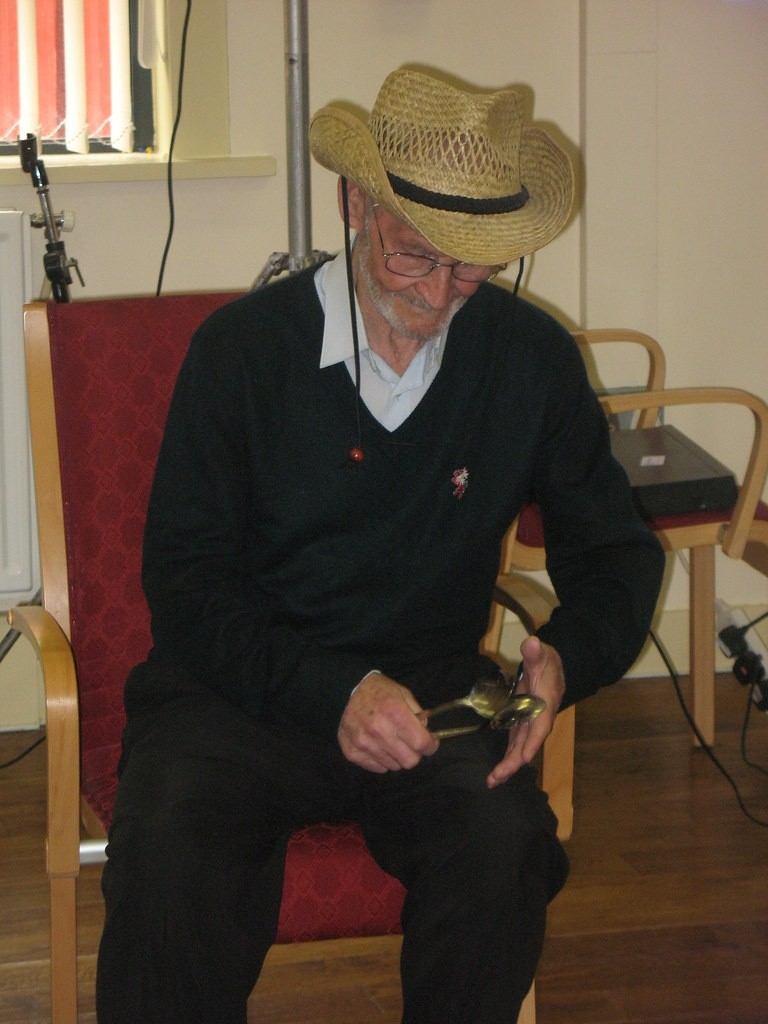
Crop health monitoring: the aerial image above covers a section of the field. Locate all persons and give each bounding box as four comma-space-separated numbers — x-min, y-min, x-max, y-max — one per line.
94, 69, 666, 1024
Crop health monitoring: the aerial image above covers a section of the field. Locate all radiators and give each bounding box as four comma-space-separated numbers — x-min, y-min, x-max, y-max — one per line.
0, 207, 43, 616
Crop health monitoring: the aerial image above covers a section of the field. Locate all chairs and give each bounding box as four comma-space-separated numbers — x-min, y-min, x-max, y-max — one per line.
4, 291, 576, 1024
509, 326, 768, 746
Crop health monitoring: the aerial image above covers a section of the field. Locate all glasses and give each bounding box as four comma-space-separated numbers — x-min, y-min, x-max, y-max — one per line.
369, 198, 509, 283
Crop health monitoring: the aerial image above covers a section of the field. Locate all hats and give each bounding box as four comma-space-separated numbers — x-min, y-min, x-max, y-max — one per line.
308, 67, 575, 266
